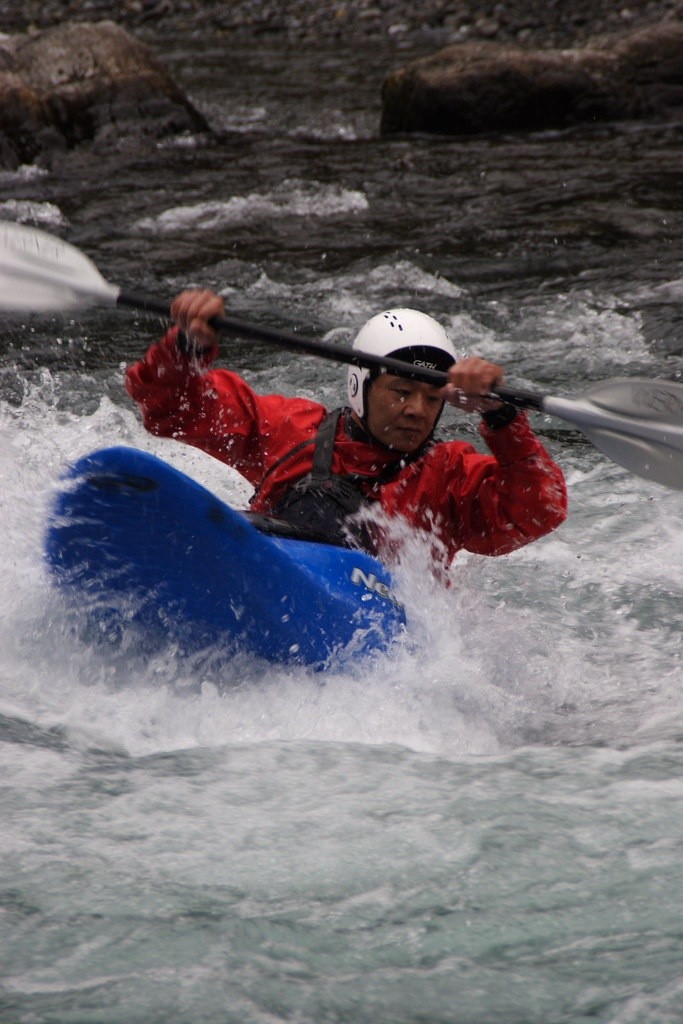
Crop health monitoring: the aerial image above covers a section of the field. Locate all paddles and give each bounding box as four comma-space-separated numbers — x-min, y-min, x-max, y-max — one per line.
1, 244, 682, 493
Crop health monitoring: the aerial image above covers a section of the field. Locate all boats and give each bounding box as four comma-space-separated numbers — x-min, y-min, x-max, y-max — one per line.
44, 446, 406, 675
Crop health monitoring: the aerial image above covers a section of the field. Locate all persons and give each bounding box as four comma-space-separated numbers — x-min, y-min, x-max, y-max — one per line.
124, 291, 568, 577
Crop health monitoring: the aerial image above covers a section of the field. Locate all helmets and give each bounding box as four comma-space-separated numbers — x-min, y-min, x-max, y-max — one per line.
347, 308, 458, 422
